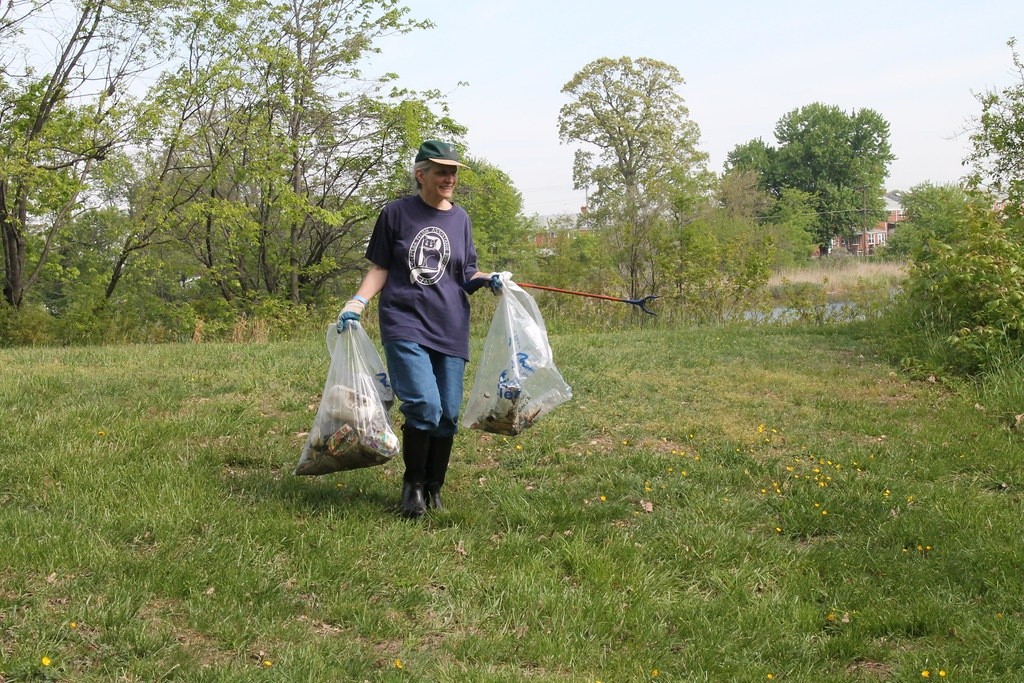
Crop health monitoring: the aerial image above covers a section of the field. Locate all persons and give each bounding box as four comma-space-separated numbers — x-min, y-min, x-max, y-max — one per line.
337, 140, 504, 514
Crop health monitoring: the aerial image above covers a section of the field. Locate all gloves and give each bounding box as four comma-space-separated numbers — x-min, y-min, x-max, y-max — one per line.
486, 271, 505, 296
336, 296, 368, 334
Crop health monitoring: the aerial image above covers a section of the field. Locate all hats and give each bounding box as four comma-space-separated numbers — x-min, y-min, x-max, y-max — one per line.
415, 140, 471, 169
